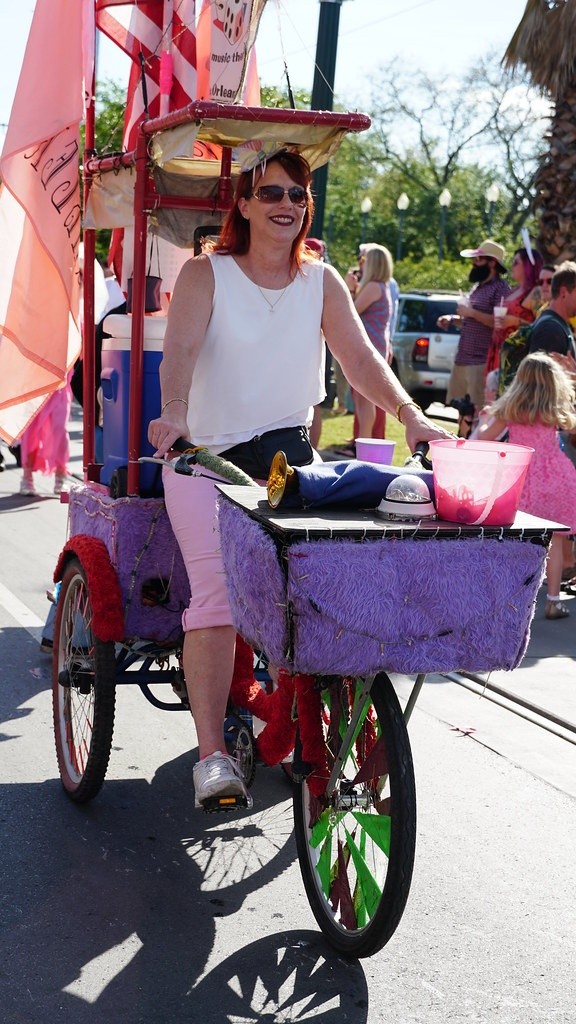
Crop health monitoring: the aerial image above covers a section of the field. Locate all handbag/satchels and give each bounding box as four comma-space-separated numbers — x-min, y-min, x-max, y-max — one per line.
128, 232, 161, 313
219, 426, 314, 480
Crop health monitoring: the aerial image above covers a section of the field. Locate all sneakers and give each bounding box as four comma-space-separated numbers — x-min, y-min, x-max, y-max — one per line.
193, 751, 246, 809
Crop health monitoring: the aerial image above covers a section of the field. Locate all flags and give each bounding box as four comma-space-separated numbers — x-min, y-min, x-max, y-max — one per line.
0, 0, 264, 445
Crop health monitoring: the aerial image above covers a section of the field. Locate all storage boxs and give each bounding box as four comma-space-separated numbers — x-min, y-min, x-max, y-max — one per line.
101, 313, 167, 491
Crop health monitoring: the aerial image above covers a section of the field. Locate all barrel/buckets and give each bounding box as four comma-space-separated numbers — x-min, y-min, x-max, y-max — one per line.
428, 439, 536, 525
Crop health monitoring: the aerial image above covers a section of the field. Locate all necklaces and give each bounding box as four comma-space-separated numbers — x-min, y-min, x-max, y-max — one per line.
242, 257, 291, 312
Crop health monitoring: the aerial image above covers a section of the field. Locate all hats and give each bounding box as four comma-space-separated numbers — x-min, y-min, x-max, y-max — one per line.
305, 238, 322, 251
461, 240, 508, 271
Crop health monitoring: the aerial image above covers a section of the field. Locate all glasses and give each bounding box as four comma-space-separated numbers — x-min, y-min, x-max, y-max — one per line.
245, 185, 308, 207
513, 259, 522, 266
536, 278, 552, 286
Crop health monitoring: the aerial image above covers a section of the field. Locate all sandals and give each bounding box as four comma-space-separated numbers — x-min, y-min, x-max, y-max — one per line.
546, 599, 570, 618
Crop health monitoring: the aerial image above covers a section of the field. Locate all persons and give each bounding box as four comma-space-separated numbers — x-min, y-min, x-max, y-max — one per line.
439, 240, 556, 440
333, 244, 400, 457
478, 354, 576, 616
148, 150, 458, 810
526, 263, 576, 586
0, 370, 71, 496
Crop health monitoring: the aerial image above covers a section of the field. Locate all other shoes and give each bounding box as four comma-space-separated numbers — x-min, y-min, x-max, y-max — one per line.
41, 638, 89, 657
54, 475, 66, 494
335, 444, 356, 457
20, 478, 36, 496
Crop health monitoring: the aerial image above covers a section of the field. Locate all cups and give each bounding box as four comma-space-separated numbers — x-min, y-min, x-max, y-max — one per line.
456, 298, 471, 318
494, 306, 508, 328
356, 437, 397, 467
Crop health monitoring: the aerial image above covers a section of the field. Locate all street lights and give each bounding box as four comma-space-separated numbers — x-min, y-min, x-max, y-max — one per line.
485, 182, 500, 239
437, 186, 453, 265
394, 191, 410, 263
357, 194, 372, 246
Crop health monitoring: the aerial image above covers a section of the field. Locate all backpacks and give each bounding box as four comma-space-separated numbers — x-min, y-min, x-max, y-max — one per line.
496, 317, 570, 398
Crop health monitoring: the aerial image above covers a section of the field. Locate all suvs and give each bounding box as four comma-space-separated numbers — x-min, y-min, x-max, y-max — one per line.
392, 288, 465, 413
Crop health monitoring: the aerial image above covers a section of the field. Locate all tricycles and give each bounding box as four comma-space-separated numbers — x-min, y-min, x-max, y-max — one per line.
50, 102, 442, 962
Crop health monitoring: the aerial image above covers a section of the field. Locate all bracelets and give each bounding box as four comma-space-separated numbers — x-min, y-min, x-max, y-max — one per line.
396, 401, 421, 423
161, 398, 189, 413
350, 290, 355, 293
